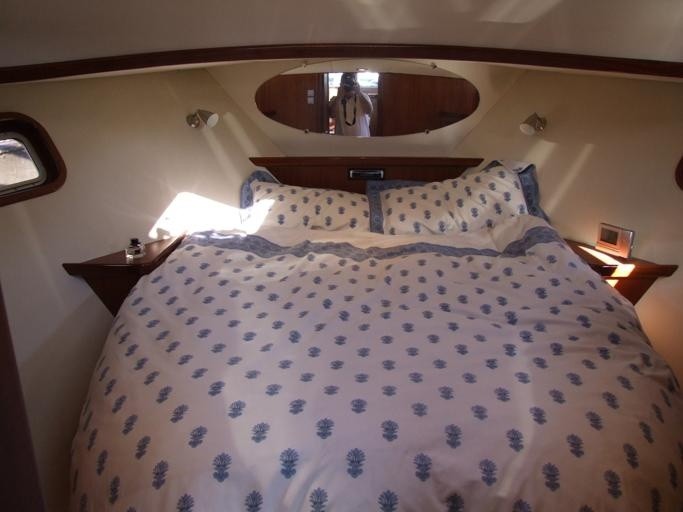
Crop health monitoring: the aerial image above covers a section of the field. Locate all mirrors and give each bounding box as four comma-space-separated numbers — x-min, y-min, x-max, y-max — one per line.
255, 59, 480, 137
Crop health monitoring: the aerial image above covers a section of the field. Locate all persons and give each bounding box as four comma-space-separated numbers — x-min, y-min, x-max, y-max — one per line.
329, 72, 374, 137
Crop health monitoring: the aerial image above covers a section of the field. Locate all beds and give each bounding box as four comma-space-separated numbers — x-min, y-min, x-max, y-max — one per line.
92, 155, 681, 511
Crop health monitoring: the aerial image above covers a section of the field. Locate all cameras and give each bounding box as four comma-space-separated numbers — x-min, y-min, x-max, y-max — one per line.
343, 73, 353, 90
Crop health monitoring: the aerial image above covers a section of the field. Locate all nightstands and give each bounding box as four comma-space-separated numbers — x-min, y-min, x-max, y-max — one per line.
565, 239, 679, 308
63, 234, 187, 316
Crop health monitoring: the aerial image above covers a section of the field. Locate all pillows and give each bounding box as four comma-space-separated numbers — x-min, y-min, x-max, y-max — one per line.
250, 179, 369, 231
368, 160, 547, 233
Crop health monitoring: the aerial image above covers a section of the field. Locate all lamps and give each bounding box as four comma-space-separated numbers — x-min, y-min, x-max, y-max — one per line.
187, 109, 219, 130
520, 113, 546, 134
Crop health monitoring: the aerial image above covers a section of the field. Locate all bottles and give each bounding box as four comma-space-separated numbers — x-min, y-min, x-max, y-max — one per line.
124, 238, 145, 259
